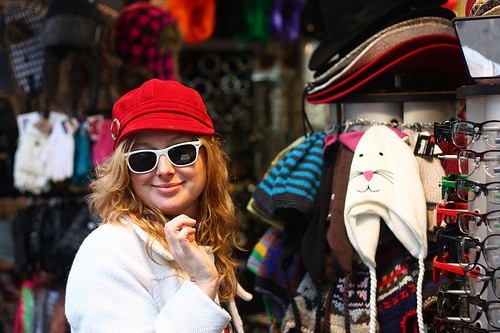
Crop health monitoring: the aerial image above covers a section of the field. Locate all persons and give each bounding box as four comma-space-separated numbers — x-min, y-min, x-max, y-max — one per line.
62, 78, 236, 333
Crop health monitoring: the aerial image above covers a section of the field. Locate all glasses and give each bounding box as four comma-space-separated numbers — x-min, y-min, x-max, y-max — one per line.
121, 138, 203, 174
434, 114, 500, 332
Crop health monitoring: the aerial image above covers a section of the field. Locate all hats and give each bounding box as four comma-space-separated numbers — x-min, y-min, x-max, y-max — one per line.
240, 125, 437, 332
302, 1, 500, 126
111, 78, 214, 149
